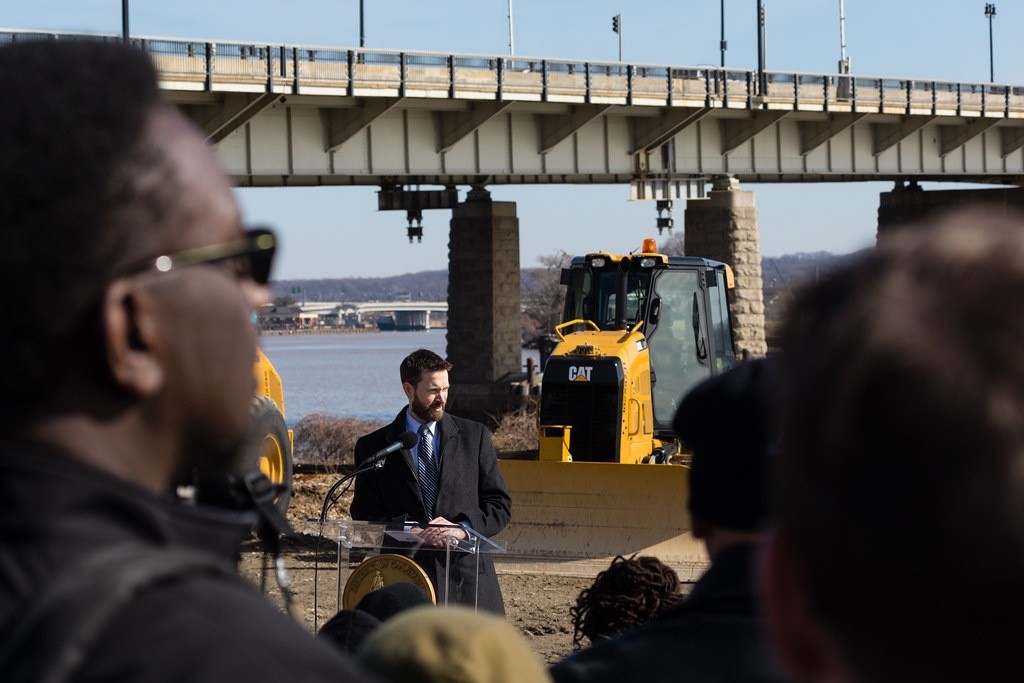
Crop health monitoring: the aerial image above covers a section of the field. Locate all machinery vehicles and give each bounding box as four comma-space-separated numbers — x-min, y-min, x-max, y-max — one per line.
195, 342, 295, 538
497, 238, 752, 584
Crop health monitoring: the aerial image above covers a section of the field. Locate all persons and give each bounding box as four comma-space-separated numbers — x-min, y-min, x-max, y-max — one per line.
546, 211, 1023, 683
316, 582, 551, 683
349, 348, 512, 624
0, 35, 363, 682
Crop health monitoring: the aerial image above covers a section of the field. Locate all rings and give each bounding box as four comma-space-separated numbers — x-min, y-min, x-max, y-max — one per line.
438, 527, 442, 532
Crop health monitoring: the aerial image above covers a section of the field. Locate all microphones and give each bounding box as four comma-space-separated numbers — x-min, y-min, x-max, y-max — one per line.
362, 432, 419, 464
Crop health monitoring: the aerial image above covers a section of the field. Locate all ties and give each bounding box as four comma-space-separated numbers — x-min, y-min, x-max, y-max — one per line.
417, 424, 437, 517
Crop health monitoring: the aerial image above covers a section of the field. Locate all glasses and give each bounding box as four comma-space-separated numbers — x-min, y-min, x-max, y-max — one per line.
136, 226, 279, 286
